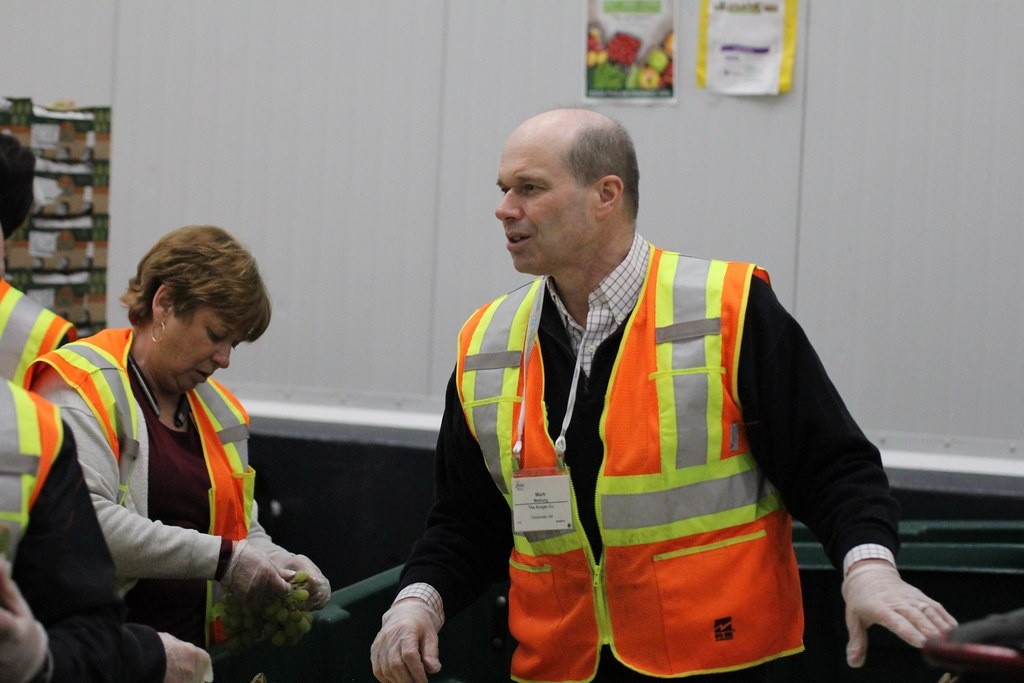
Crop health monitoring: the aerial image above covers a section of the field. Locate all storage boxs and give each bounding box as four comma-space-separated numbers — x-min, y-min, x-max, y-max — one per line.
0, 96, 110, 339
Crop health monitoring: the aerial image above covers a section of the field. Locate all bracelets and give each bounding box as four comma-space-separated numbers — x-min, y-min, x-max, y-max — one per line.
841, 563, 900, 598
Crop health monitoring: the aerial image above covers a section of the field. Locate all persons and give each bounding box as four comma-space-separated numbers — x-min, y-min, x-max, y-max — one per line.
369, 108, 959, 683
24, 225, 331, 652
0, 378, 121, 682
0, 131, 76, 393
120, 621, 213, 683
